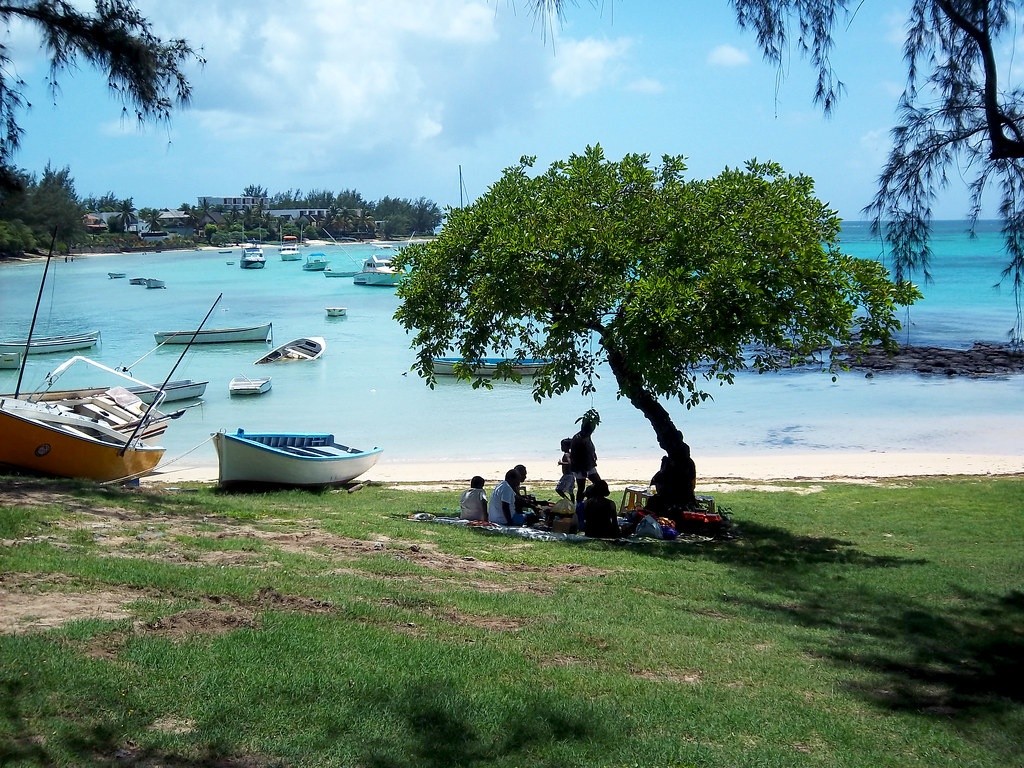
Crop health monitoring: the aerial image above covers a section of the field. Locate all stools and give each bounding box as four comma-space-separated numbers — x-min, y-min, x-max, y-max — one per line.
618, 487, 646, 513
695, 495, 716, 512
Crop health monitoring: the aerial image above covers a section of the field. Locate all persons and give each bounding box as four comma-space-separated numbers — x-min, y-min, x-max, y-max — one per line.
585, 480, 623, 538
576, 485, 594, 531
514, 465, 526, 482
490, 469, 535, 527
571, 419, 601, 502
556, 439, 575, 504
460, 476, 488, 522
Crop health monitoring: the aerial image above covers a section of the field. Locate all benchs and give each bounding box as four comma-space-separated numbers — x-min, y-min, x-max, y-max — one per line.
286, 346, 311, 358
287, 445, 328, 456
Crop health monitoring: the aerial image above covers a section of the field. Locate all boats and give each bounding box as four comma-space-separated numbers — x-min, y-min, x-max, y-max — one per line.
226, 260, 235, 266
1, 329, 105, 356
323, 270, 359, 277
251, 337, 326, 367
427, 357, 558, 377
153, 322, 273, 343
302, 252, 331, 271
107, 271, 125, 279
129, 277, 145, 286
322, 227, 415, 286
0, 224, 223, 481
326, 306, 347, 317
278, 228, 302, 260
229, 375, 273, 395
374, 243, 394, 251
241, 238, 267, 269
118, 379, 209, 402
0, 353, 23, 370
208, 428, 385, 488
146, 278, 164, 288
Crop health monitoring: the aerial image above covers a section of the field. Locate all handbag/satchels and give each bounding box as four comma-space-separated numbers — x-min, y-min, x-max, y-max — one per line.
635, 509, 678, 539
